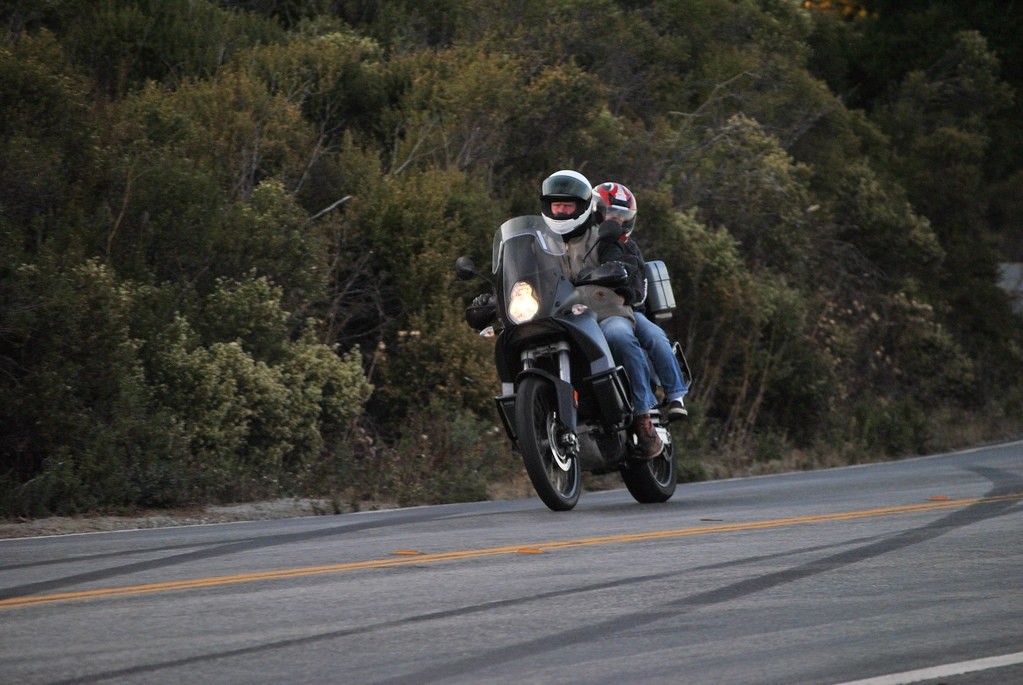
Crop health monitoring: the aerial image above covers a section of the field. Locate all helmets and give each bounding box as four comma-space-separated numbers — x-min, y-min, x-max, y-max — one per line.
541, 170, 592, 235
592, 182, 637, 243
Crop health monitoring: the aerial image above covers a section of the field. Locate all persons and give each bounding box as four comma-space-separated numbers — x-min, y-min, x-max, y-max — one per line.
592, 182, 688, 415
472, 168, 664, 458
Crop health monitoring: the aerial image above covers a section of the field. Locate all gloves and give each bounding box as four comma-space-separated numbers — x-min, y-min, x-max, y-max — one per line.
590, 262, 625, 286
467, 293, 496, 317
617, 286, 637, 307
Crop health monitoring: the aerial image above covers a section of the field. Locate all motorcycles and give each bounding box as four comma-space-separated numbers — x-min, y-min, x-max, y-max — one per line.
453, 215, 695, 512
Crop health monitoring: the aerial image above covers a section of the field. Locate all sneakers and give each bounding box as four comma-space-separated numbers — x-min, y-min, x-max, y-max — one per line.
668, 399, 688, 417
632, 415, 665, 459
